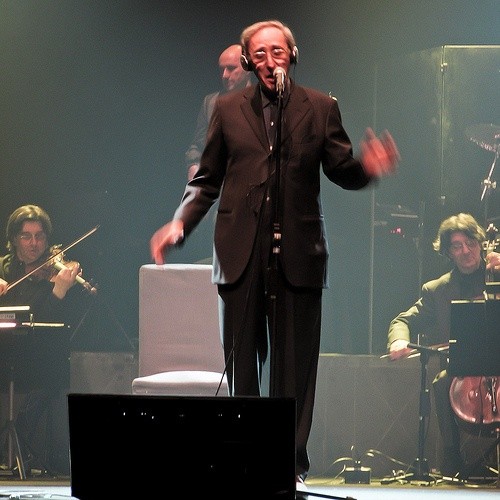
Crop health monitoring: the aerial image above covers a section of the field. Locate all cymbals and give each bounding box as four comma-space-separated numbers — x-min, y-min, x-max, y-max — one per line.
464, 123, 500, 152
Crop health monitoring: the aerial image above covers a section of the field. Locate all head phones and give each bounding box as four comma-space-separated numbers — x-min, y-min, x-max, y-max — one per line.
239, 43, 299, 71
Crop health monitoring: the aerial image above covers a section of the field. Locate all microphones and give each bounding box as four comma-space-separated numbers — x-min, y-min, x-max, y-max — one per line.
272, 67, 287, 92
407, 344, 443, 354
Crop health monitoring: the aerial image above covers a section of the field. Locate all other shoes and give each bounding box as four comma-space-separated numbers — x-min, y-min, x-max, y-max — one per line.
296, 473, 308, 483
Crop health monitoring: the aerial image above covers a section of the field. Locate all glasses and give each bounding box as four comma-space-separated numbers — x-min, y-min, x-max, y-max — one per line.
451, 238, 477, 251
17, 231, 45, 241
247, 49, 290, 63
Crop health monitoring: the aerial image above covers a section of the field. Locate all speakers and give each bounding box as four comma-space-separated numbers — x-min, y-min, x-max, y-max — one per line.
66, 391, 297, 500
69, 353, 138, 393
313, 351, 439, 483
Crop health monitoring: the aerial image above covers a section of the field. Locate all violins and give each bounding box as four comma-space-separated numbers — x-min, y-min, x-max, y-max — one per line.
24, 248, 97, 295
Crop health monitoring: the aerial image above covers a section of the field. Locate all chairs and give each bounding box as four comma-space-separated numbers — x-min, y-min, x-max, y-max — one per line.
131, 264, 229, 396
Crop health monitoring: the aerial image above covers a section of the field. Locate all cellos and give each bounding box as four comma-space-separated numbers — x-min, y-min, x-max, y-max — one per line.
450, 223, 500, 485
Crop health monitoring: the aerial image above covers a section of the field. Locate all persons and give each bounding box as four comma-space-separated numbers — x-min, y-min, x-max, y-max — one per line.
389, 214, 500, 478
186, 45, 253, 221
151, 20, 400, 486
0, 205, 82, 477
